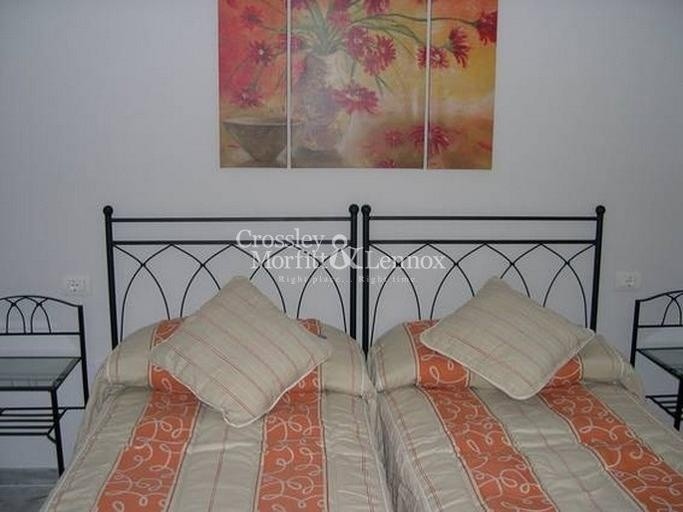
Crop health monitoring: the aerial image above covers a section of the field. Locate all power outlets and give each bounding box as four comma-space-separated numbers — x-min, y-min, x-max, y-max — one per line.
63, 274, 90, 296
613, 269, 641, 291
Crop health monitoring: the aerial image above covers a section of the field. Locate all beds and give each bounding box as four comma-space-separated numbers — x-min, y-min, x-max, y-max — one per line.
361, 205, 683, 512
39, 204, 396, 512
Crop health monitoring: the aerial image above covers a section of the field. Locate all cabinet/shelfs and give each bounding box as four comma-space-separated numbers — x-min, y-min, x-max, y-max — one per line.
0, 296, 88, 477
630, 291, 683, 431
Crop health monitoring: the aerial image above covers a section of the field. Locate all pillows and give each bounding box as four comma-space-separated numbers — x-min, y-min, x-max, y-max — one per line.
371, 319, 624, 392
107, 316, 364, 396
146, 274, 334, 427
419, 277, 596, 401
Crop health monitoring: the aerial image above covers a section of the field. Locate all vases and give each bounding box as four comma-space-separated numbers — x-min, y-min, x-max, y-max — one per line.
283, 54, 351, 151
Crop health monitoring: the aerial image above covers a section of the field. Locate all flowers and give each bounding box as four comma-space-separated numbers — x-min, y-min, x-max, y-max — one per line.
226, 0, 498, 118
